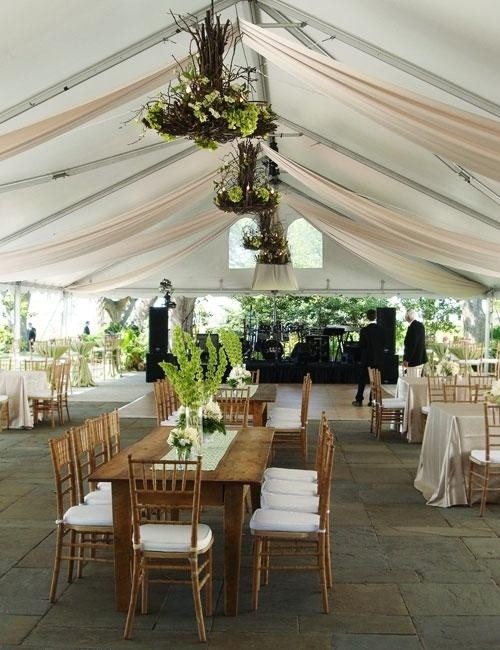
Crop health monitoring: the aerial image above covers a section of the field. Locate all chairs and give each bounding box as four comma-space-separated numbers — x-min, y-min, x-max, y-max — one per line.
264, 371, 322, 469
151, 375, 186, 426
123, 453, 218, 644
367, 365, 406, 440
45, 406, 137, 603
0, 357, 73, 432
248, 408, 335, 613
463, 402, 500, 518
418, 372, 495, 402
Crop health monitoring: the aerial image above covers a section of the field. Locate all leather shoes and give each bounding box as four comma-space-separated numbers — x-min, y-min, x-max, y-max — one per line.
352, 401, 362, 407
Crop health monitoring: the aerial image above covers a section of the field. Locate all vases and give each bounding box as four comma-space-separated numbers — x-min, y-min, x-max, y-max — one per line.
175, 446, 190, 470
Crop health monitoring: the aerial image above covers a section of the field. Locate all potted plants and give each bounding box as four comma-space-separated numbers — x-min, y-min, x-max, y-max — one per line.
210, 326, 251, 396
159, 326, 230, 470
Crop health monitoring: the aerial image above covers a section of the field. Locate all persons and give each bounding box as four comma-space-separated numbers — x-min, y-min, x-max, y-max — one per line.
352, 309, 385, 406
84, 321, 91, 335
403, 309, 429, 377
26, 322, 36, 345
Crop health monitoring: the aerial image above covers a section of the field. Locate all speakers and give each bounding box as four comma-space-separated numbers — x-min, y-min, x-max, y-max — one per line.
148, 306, 168, 350
377, 306, 396, 354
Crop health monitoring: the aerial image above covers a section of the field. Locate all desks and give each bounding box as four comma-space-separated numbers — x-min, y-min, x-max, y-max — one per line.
409, 401, 499, 508
394, 371, 462, 441
85, 421, 278, 617
217, 380, 279, 431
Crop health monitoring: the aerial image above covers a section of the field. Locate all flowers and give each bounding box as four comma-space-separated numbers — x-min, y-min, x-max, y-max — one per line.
177, 402, 227, 435
166, 427, 198, 447
226, 366, 251, 387
